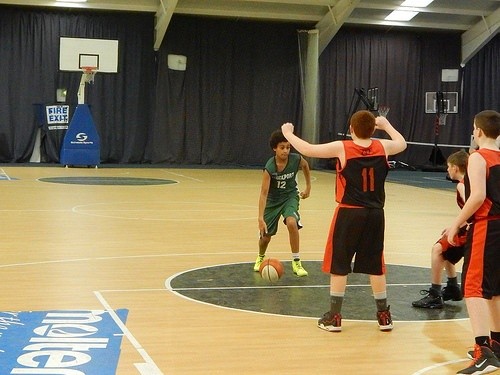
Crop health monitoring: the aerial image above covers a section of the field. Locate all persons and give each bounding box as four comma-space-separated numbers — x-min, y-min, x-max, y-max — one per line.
280, 110, 407, 332
447, 110, 500, 375
412, 150, 469, 308
253, 130, 311, 277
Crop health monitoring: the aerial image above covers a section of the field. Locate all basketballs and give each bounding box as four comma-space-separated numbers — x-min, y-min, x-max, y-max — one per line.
260, 258, 284, 283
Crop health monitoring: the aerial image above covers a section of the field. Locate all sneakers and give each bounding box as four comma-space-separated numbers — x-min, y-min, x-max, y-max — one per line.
467, 339, 500, 361
412, 288, 443, 309
317, 311, 341, 331
377, 305, 394, 330
254, 256, 265, 271
442, 281, 463, 301
291, 258, 308, 277
456, 344, 500, 375
